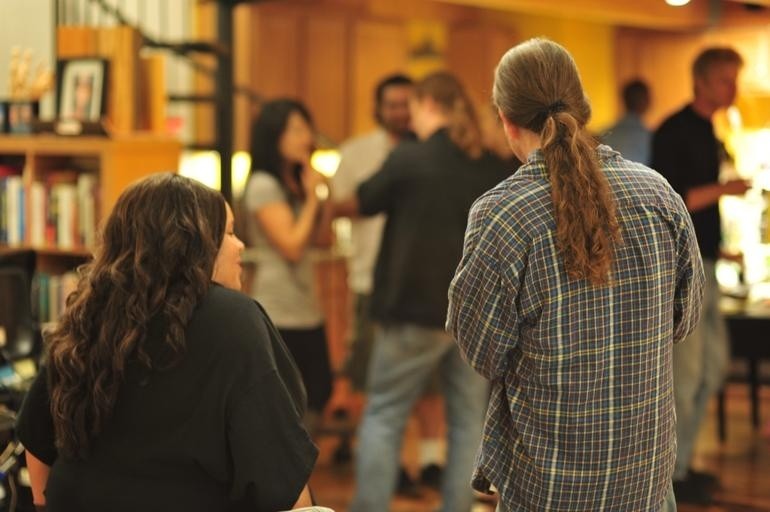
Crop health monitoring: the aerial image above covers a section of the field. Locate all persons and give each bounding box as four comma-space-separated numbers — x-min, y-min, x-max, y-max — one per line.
16, 171, 322, 511
653, 46, 753, 505
332, 70, 519, 511
597, 81, 655, 166
241, 95, 336, 442
445, 37, 708, 511
328, 75, 447, 499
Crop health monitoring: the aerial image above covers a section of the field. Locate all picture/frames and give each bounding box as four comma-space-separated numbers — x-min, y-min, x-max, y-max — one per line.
56, 54, 107, 136
0, 99, 39, 136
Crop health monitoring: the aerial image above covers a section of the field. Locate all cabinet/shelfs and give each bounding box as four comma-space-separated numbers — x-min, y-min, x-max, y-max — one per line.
1, 139, 179, 333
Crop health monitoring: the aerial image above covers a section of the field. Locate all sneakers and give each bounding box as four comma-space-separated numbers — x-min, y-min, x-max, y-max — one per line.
672, 472, 719, 503
395, 462, 443, 497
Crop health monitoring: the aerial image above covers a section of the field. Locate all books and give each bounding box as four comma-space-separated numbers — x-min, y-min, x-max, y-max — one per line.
0, 168, 98, 331
